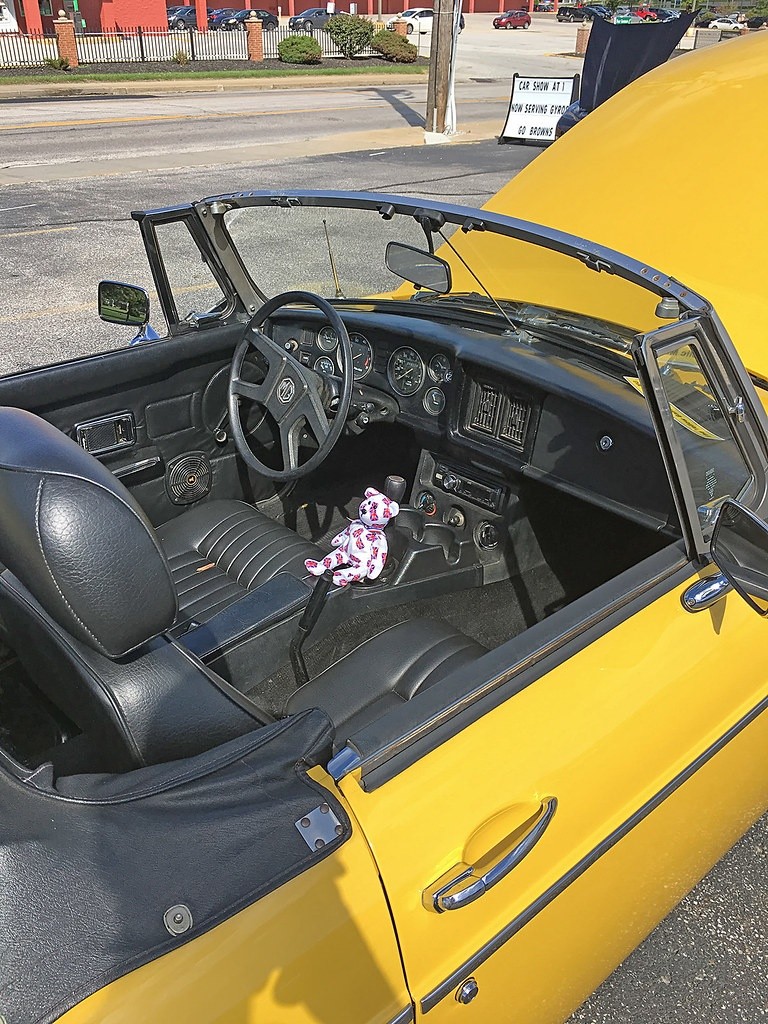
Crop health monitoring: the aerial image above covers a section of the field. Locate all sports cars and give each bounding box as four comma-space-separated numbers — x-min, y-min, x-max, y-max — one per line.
0, 28, 768, 1024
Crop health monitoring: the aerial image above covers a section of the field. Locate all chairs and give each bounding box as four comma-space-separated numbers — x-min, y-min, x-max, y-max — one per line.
152, 498, 349, 695
0, 407, 489, 771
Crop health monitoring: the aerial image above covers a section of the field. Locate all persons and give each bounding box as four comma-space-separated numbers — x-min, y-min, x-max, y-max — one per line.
578, 0, 587, 8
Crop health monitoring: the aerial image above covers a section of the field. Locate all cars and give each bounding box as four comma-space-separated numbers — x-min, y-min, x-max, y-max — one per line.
708, 17, 745, 30
386, 7, 434, 35
289, 7, 347, 32
493, 10, 532, 30
208, 8, 241, 31
695, 17, 717, 28
556, 3, 681, 25
220, 8, 279, 32
166, 5, 215, 30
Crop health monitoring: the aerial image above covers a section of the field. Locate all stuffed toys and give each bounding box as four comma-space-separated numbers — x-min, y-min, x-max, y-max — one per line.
304, 487, 399, 587
